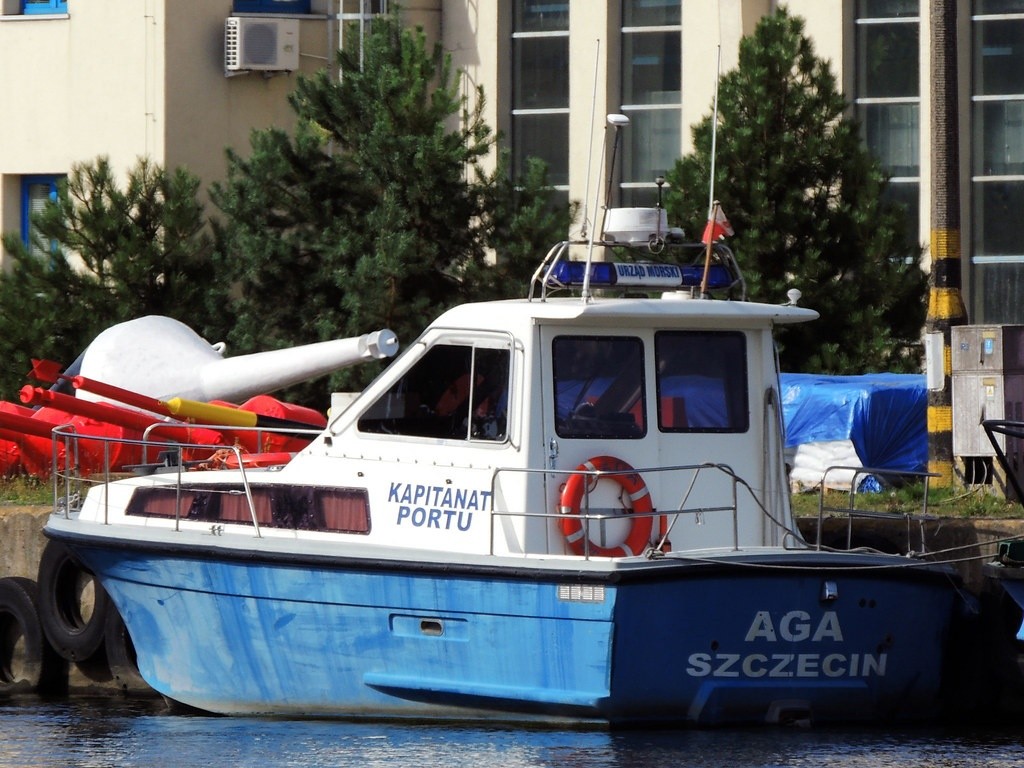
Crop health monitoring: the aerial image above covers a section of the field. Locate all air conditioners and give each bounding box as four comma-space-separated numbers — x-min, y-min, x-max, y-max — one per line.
224, 17, 300, 71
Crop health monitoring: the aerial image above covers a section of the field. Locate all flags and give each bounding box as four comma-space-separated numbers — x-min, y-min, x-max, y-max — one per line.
701, 204, 733, 245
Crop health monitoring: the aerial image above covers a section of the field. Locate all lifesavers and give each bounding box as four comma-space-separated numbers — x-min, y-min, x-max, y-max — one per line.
558, 453, 655, 556
106, 600, 160, 701
0, 576, 69, 692
35, 538, 112, 661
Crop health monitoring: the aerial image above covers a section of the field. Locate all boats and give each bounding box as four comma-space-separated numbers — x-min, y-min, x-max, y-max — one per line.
44, 39, 1024, 726
